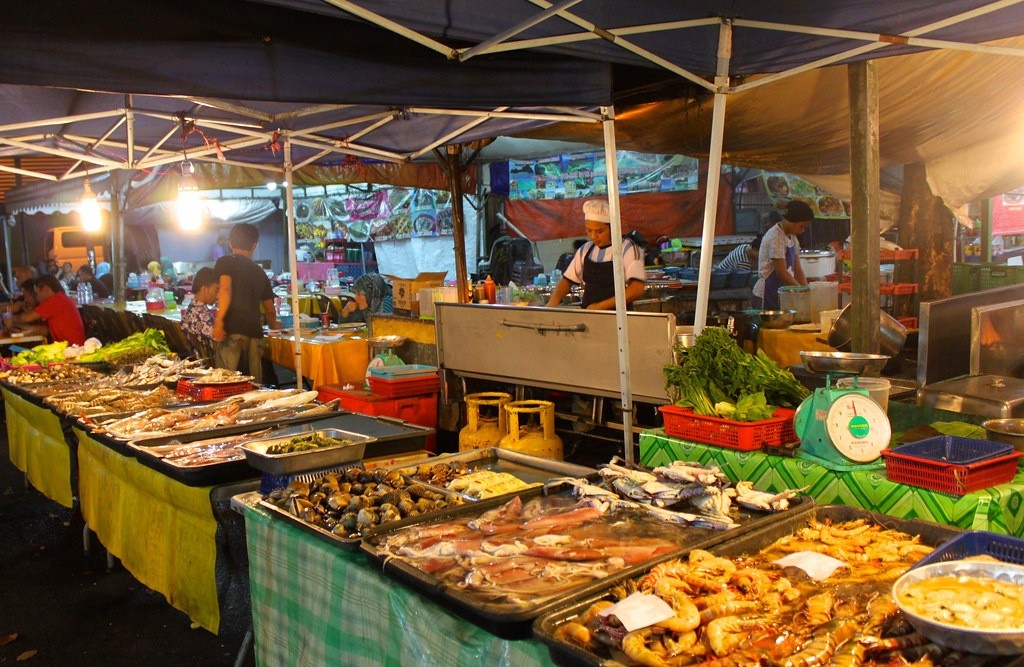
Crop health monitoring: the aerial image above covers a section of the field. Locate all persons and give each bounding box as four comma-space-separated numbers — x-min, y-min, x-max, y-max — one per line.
180, 267, 221, 356
0, 257, 113, 347
212, 222, 283, 384
125, 260, 200, 301
544, 199, 646, 441
555, 230, 669, 275
716, 234, 762, 270
339, 272, 394, 322
751, 200, 814, 327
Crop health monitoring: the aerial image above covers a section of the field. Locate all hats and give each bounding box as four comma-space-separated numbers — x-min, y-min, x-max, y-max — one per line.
782, 201, 813, 222
583, 200, 610, 222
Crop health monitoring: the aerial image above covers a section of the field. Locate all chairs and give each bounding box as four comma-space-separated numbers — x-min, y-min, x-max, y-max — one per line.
476, 236, 544, 286
83, 304, 191, 359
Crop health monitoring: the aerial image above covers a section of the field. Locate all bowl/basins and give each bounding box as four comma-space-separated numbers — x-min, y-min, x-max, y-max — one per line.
981, 417, 1024, 457
756, 309, 797, 329
891, 559, 1024, 655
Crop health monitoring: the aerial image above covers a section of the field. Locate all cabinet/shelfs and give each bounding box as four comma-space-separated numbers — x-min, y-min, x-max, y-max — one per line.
838, 248, 919, 333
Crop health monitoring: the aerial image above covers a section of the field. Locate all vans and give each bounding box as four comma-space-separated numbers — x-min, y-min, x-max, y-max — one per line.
46, 226, 104, 273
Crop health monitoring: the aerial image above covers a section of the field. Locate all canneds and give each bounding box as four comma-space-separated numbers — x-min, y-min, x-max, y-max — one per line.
321, 313, 329, 327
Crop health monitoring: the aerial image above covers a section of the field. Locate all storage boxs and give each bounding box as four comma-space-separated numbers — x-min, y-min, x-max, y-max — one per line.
879, 434, 1024, 496
659, 404, 797, 450
778, 281, 838, 324
951, 262, 1024, 295
419, 286, 459, 318
905, 530, 1024, 582
377, 270, 447, 317
366, 363, 441, 398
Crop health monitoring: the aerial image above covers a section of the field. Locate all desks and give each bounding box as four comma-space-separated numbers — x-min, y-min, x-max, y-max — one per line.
0, 330, 45, 357
744, 323, 839, 369
296, 261, 377, 283
638, 401, 1024, 538
669, 235, 758, 268
99, 299, 370, 390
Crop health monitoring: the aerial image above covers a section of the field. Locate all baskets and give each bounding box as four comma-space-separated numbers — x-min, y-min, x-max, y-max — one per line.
176, 380, 253, 400
256, 461, 366, 495
367, 363, 440, 398
881, 435, 1024, 495
903, 529, 1024, 567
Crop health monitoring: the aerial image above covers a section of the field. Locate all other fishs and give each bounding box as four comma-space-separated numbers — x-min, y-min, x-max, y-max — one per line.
30, 340, 343, 471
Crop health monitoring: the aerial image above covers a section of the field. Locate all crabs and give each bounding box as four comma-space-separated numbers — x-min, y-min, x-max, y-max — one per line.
540, 456, 812, 530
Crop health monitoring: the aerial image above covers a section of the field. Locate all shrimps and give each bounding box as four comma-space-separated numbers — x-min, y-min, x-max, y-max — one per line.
546, 516, 980, 667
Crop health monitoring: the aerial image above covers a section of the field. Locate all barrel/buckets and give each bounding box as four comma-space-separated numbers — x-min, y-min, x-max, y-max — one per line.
827, 302, 908, 371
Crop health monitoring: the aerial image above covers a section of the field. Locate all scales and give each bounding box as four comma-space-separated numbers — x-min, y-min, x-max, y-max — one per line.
793, 351, 893, 472
364, 333, 407, 390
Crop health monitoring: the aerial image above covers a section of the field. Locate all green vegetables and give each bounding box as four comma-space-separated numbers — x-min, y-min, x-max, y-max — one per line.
658, 326, 814, 422
6, 326, 170, 366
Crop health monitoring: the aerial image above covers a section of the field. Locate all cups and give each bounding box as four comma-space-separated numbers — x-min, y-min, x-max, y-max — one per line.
2, 312, 14, 330
321, 313, 329, 327
837, 376, 891, 417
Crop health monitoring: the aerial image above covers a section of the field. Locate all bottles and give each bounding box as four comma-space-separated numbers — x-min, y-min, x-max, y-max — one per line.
550, 270, 563, 286
474, 274, 497, 304
76, 281, 94, 304
127, 272, 149, 289
272, 296, 291, 316
145, 286, 166, 315
